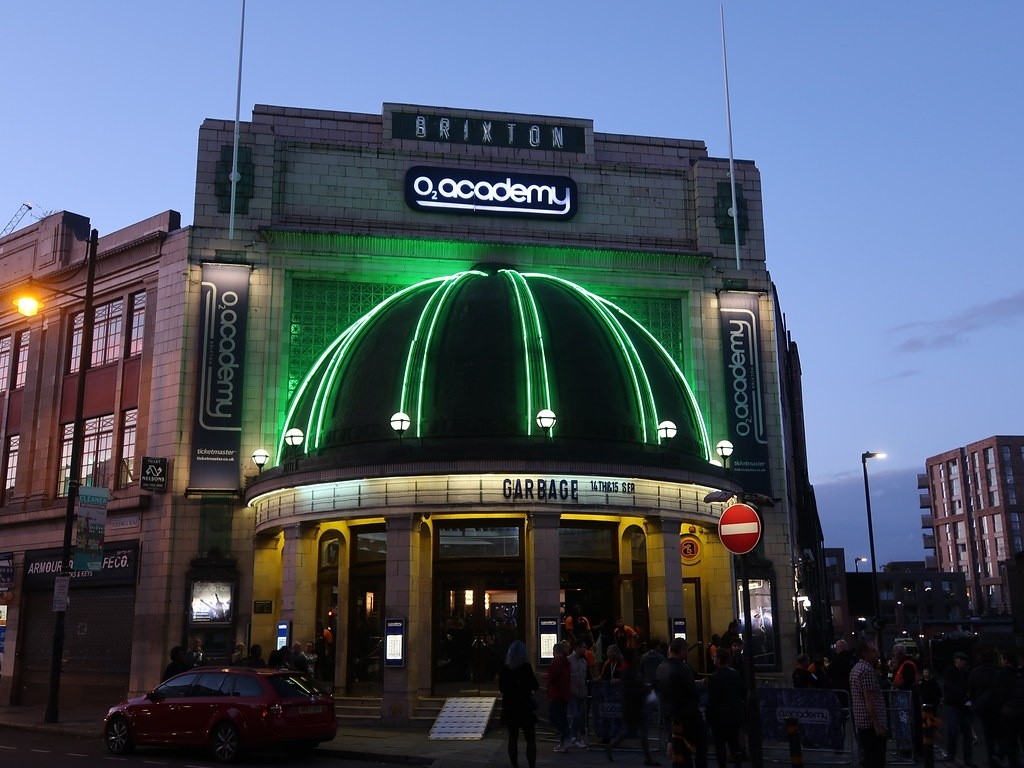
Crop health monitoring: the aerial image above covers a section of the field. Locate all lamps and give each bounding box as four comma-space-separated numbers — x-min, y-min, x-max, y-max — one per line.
284, 428, 304, 470
658, 420, 678, 448
535, 409, 557, 437
251, 449, 270, 474
389, 411, 411, 446
715, 440, 734, 468
703, 490, 735, 504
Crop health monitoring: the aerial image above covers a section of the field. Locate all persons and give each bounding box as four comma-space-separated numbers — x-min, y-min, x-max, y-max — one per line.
439, 601, 1024, 768
160, 636, 318, 682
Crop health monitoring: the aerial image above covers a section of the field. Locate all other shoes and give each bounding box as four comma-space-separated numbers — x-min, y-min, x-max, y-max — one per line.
572, 738, 585, 749
553, 741, 572, 752
644, 761, 661, 766
606, 746, 613, 762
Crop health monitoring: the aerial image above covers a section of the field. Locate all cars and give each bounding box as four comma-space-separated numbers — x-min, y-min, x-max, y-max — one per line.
893, 635, 946, 674
102, 665, 338, 766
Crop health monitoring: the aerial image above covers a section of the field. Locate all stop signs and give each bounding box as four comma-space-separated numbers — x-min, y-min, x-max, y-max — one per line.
718, 503, 761, 555
855, 557, 867, 574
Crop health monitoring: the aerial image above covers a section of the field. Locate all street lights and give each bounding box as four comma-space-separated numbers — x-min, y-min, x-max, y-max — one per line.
16, 228, 100, 726
861, 450, 892, 740
